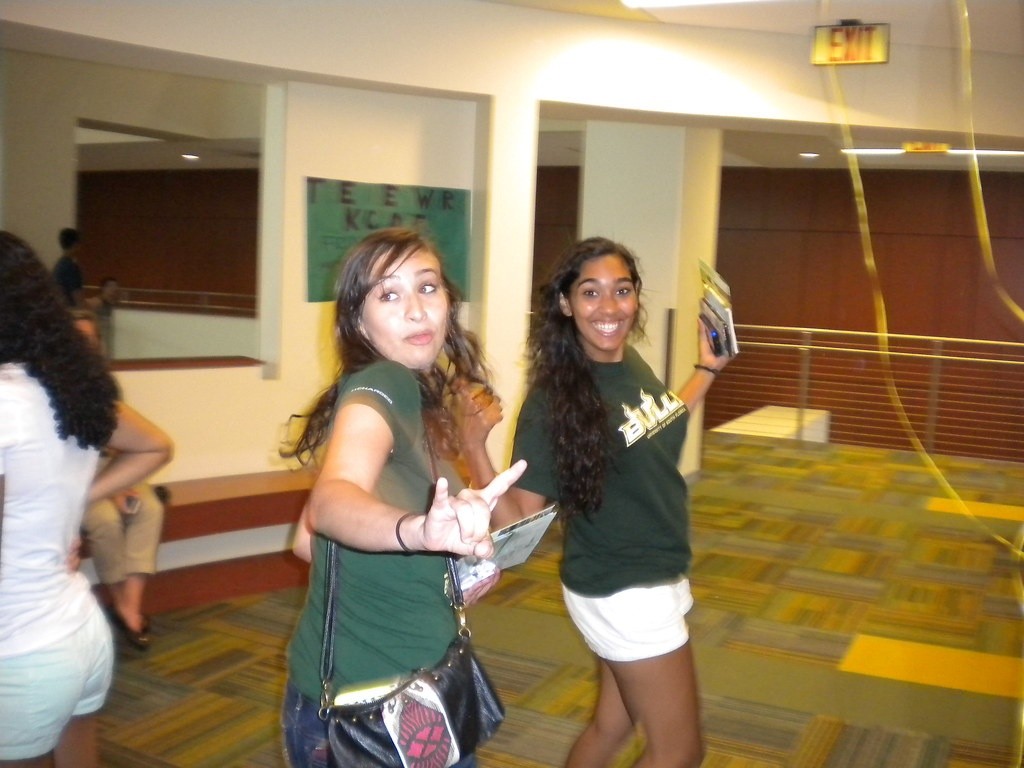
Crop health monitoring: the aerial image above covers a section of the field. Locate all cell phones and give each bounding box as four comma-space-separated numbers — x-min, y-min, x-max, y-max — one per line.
699, 313, 722, 357
126, 496, 141, 514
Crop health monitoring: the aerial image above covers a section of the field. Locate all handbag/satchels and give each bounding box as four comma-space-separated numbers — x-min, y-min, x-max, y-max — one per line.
319, 636, 505, 768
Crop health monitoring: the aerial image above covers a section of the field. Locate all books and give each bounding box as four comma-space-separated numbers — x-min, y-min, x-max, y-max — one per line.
704, 285, 739, 354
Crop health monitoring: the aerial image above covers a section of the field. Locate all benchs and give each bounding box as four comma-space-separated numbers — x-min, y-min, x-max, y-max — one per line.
75, 464, 321, 624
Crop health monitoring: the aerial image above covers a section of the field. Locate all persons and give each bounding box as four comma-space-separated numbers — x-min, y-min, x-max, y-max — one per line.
0, 231, 176, 767
449, 235, 736, 768
53, 229, 119, 360
67, 313, 167, 646
282, 229, 528, 768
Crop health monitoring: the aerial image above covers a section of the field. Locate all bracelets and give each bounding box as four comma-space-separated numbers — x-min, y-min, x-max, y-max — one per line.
469, 472, 499, 488
695, 365, 719, 375
396, 512, 416, 553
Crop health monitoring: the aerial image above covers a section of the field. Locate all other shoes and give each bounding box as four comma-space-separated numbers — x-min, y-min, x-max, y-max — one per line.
105, 605, 149, 649
